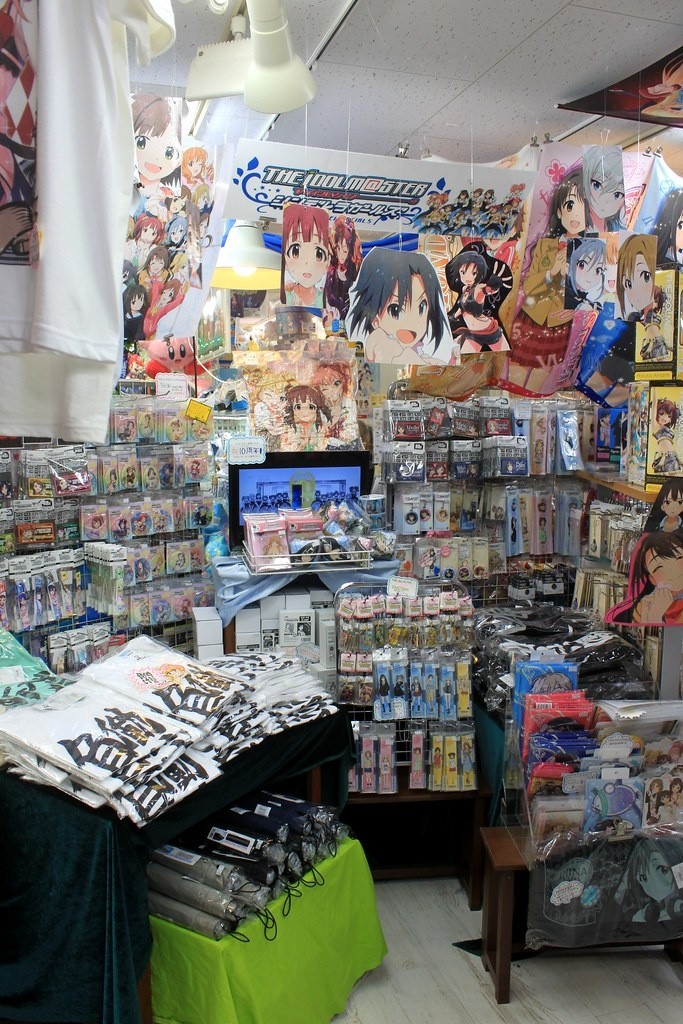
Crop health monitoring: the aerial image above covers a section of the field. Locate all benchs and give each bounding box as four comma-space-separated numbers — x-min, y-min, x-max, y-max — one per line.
475, 823, 683, 1004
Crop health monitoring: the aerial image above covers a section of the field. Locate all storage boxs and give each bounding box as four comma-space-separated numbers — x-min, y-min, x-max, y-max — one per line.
148, 836, 387, 1024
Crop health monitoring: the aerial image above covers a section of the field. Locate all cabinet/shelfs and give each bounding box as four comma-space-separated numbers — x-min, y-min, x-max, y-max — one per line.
1, 704, 351, 1024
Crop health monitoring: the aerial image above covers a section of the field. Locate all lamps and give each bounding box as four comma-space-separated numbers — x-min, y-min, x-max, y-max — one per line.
240, 0, 318, 116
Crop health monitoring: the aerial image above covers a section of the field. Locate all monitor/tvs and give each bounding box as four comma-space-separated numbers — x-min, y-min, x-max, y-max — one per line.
228, 451, 374, 552
